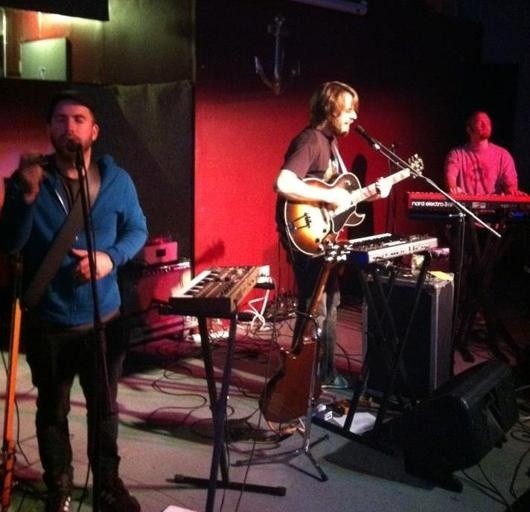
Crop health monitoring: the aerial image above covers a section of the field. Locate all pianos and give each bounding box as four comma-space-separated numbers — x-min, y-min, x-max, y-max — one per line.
338, 233, 439, 268
406, 192, 530, 217
169, 264, 261, 312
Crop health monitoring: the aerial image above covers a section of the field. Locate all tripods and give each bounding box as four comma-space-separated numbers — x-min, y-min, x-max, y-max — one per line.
237, 335, 331, 480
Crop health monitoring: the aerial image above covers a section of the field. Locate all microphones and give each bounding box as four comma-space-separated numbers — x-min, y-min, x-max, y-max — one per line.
355, 124, 381, 150
65, 136, 83, 153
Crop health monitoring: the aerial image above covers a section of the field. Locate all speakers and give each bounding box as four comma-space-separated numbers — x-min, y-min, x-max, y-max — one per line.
122, 259, 196, 346
365, 275, 453, 393
379, 357, 519, 472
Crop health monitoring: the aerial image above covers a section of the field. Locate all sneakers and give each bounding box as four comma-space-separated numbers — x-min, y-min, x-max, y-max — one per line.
318, 367, 362, 389
454, 340, 476, 363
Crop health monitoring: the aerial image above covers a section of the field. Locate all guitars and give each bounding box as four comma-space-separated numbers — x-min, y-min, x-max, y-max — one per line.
284, 155, 424, 257
259, 237, 342, 422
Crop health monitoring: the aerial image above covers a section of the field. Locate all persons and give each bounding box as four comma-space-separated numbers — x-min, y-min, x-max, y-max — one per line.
271, 79, 393, 418
442, 109, 521, 362
1, 88, 151, 512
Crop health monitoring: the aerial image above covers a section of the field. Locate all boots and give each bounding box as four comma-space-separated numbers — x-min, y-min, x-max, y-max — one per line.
86, 455, 141, 512
39, 460, 76, 512
